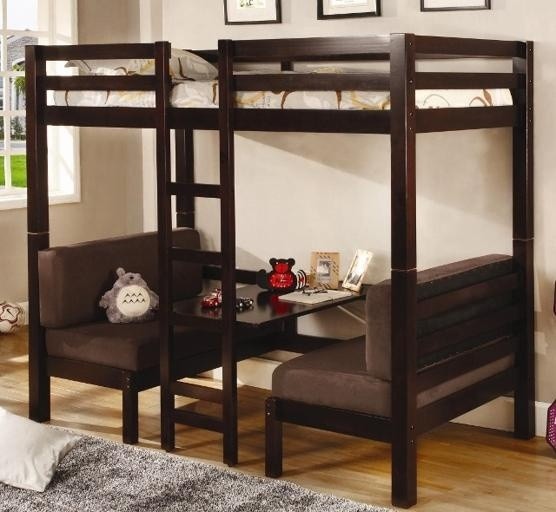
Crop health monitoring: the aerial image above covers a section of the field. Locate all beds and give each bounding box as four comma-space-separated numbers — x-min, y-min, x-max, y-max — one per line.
25, 30, 539, 510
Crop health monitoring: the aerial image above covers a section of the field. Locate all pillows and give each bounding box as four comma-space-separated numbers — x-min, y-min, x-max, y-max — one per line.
0, 405, 83, 492
61, 45, 222, 83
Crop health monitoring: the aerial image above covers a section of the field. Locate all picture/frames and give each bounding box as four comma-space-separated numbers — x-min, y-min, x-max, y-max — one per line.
313, 0, 385, 20
342, 247, 375, 293
221, 0, 284, 27
416, 0, 492, 14
309, 249, 341, 289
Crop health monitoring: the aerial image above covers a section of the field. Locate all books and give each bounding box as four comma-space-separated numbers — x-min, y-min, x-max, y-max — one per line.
278, 287, 353, 305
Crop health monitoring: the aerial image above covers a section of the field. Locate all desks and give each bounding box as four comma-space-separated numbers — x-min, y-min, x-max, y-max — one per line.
159, 271, 376, 467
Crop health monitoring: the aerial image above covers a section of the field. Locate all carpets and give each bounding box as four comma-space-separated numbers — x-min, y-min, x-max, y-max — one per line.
0, 410, 398, 510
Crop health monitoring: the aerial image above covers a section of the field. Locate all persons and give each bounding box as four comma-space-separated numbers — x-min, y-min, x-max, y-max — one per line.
317, 262, 330, 276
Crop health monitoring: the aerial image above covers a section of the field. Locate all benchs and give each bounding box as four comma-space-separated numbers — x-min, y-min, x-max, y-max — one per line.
27, 226, 286, 451
258, 247, 539, 507
